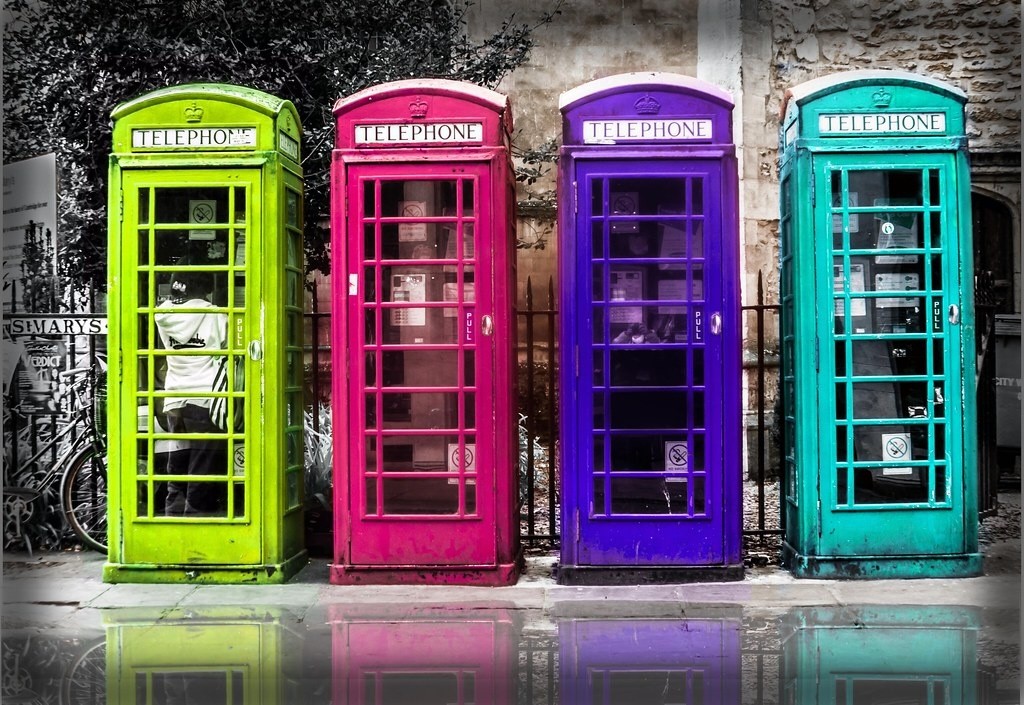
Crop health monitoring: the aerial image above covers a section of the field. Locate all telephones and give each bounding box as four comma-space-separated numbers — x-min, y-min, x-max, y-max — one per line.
389, 273, 428, 328
611, 265, 646, 326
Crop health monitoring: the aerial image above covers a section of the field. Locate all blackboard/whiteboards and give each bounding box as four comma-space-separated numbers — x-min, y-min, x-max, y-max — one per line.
833, 256, 872, 335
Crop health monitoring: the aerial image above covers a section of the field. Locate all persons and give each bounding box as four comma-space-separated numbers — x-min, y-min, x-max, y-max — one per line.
154, 254, 228, 517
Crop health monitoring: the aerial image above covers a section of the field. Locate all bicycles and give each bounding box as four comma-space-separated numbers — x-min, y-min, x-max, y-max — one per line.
1, 363, 107, 556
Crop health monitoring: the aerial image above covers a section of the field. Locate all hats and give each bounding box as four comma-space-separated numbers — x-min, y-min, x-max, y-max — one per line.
169, 254, 215, 296
